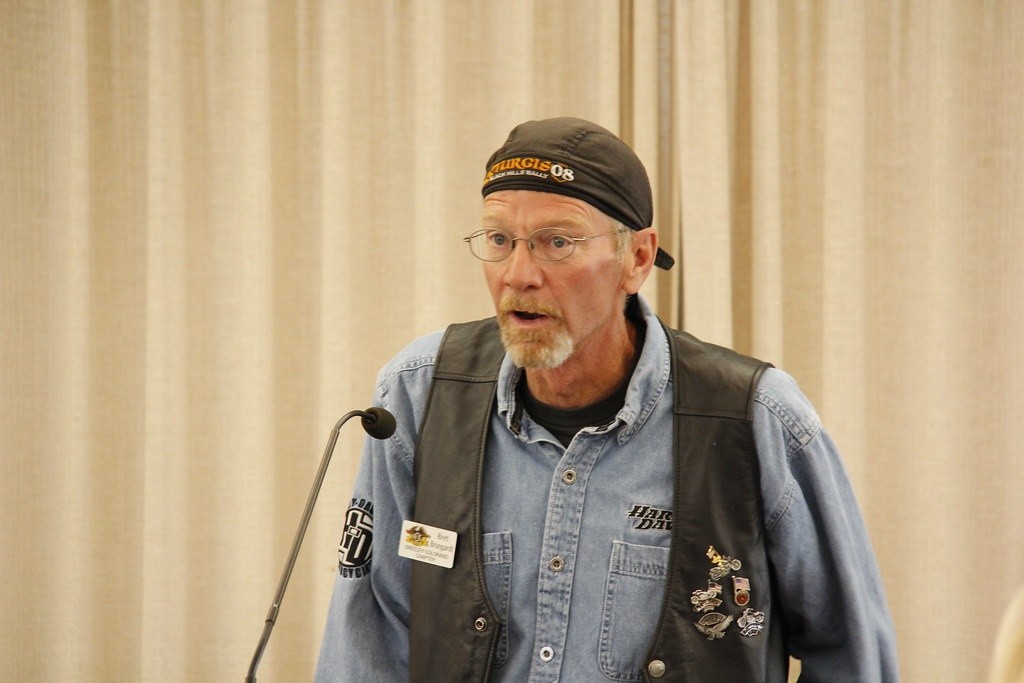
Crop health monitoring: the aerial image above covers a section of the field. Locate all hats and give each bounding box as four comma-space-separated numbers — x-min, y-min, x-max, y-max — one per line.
482, 116, 675, 271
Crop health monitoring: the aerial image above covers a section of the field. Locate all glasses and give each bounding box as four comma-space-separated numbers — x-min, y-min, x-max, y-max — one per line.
464, 228, 630, 262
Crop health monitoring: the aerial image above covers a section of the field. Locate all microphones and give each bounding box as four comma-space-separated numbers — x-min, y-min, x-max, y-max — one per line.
244, 407, 397, 683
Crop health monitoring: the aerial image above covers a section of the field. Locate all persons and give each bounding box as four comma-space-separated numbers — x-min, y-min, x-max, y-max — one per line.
315, 116, 898, 683
